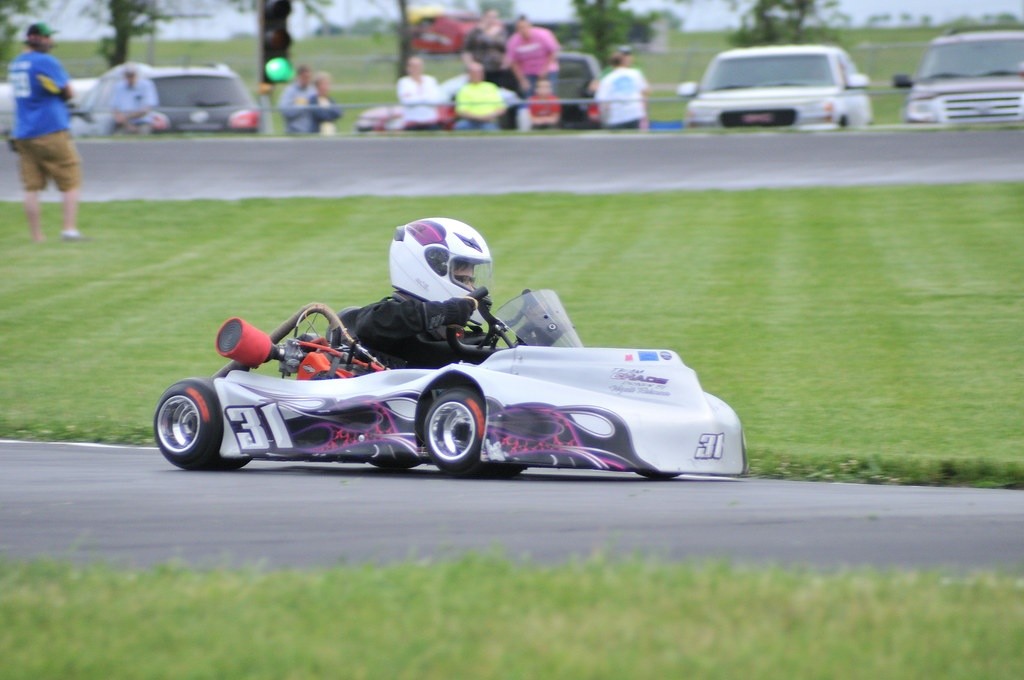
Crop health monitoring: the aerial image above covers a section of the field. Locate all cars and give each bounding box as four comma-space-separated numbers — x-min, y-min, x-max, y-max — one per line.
892, 30, 1024, 125
678, 45, 872, 128
356, 51, 603, 133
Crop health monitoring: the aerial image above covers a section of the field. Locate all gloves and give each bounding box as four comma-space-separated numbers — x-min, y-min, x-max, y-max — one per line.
423, 297, 476, 330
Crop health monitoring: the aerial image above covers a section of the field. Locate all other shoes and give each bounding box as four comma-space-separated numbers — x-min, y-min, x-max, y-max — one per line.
60, 234, 93, 243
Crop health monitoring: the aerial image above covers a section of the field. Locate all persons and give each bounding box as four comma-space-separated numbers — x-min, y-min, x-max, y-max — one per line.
394, 55, 449, 132
588, 45, 655, 131
275, 64, 345, 136
5, 22, 86, 245
108, 63, 160, 138
452, 7, 562, 131
354, 217, 574, 370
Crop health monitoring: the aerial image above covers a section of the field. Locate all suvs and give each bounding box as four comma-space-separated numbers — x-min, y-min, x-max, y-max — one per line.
67, 61, 261, 136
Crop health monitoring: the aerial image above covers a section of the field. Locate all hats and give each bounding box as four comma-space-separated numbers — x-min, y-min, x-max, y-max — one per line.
617, 45, 634, 54
28, 24, 58, 36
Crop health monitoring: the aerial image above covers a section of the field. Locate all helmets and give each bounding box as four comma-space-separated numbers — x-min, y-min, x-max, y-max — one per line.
389, 218, 492, 326
